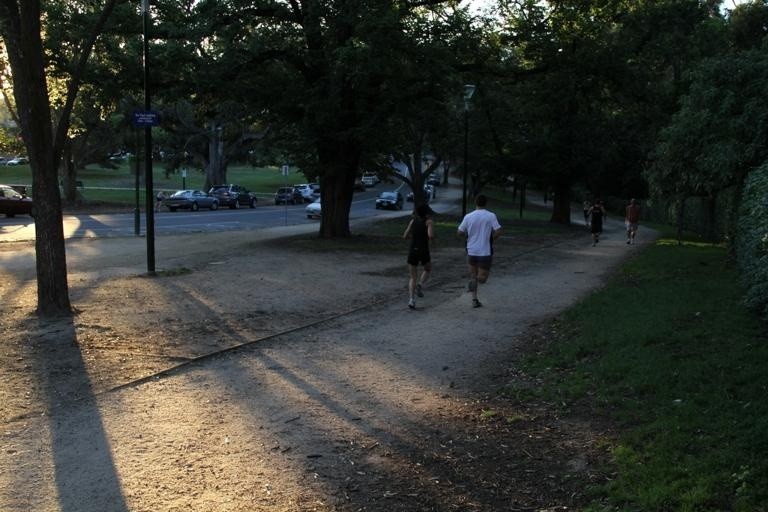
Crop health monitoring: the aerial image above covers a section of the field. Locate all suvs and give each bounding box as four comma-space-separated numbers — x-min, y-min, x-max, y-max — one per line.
209, 183, 258, 210
275, 183, 321, 218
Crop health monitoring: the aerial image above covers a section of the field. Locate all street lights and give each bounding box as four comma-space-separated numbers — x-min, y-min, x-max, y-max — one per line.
458, 84, 477, 220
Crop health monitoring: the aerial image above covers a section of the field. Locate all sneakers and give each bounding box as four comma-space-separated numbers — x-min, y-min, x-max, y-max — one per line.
471, 299, 483, 308
467, 278, 477, 293
407, 298, 417, 310
414, 281, 425, 298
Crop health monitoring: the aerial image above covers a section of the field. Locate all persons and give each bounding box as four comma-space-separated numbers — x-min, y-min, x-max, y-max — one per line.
599, 201, 604, 206
402, 203, 434, 310
587, 199, 607, 247
624, 198, 640, 245
456, 193, 502, 307
583, 201, 592, 227
154, 191, 169, 212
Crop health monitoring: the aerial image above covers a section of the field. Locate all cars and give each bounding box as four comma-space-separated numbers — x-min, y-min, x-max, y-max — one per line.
0, 154, 29, 167
403, 155, 440, 204
0, 183, 34, 219
165, 189, 220, 212
366, 169, 379, 182
375, 191, 404, 211
353, 179, 365, 192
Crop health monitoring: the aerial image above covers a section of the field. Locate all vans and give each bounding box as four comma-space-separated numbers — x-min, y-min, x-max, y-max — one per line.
382, 153, 393, 163
360, 173, 375, 187
